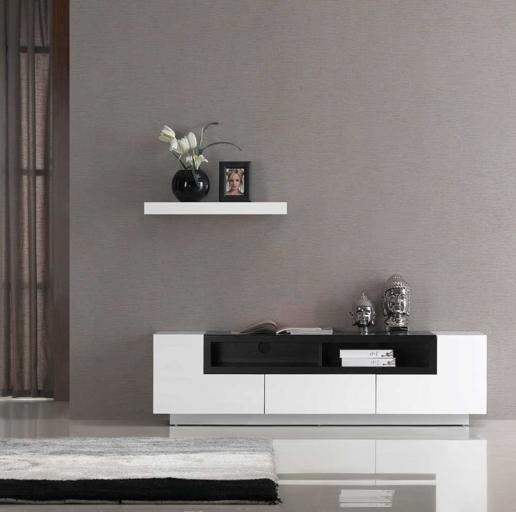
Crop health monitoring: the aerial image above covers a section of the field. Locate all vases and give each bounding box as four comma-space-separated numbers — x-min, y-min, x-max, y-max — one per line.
170, 167, 210, 203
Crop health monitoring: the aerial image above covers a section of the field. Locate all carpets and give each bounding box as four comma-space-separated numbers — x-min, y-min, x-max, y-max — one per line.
0, 433, 278, 506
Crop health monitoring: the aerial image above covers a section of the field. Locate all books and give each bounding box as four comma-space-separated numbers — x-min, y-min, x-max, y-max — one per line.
339, 348, 394, 359
339, 487, 397, 509
286, 328, 334, 336
236, 320, 322, 336
341, 357, 396, 367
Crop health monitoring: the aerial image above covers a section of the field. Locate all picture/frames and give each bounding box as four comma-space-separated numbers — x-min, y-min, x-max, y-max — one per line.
218, 159, 250, 203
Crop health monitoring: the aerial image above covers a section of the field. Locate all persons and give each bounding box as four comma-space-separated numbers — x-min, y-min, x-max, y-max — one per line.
382, 274, 412, 328
348, 289, 377, 335
224, 168, 246, 196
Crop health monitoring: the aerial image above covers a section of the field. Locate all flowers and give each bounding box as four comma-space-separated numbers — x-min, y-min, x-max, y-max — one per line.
157, 119, 240, 169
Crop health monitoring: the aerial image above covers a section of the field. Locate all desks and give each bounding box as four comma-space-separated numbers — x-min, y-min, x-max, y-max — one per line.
152, 329, 488, 427
